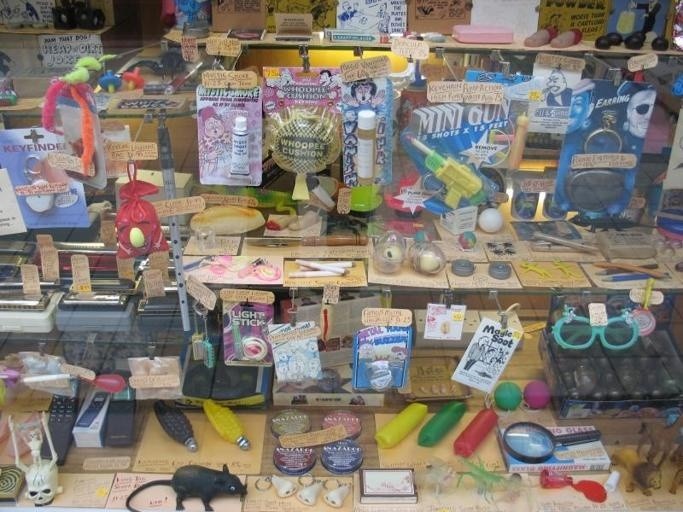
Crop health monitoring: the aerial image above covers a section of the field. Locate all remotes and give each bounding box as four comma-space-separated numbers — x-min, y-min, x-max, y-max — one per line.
41, 393, 78, 466
77, 391, 107, 427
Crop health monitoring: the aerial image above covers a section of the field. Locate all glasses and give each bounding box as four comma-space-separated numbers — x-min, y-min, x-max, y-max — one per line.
553, 306, 639, 350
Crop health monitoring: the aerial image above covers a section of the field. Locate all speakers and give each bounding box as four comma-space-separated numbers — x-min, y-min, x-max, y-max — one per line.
53, 7, 76, 29
76, 8, 105, 29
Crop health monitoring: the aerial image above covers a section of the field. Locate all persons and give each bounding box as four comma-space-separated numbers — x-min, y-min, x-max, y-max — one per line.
268, 69, 381, 113
544, 65, 573, 110
200, 107, 234, 180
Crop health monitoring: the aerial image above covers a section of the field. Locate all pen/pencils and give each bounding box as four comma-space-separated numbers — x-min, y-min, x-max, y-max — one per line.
594, 261, 672, 281
288, 259, 356, 278
